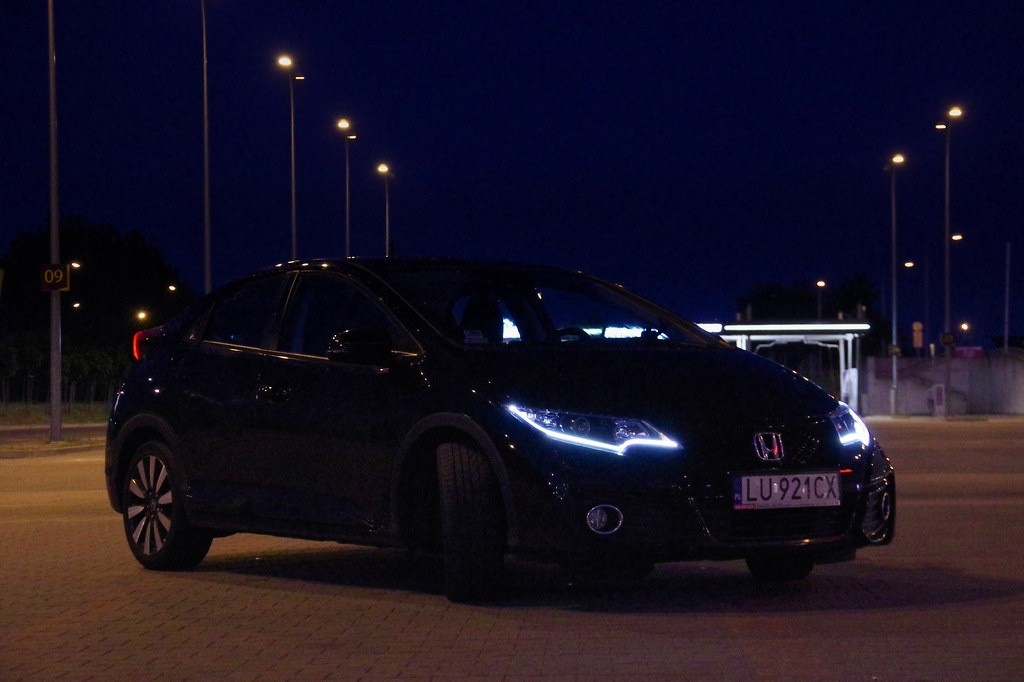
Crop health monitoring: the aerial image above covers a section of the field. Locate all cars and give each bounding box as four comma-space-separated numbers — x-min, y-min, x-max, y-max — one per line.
104, 256, 898, 606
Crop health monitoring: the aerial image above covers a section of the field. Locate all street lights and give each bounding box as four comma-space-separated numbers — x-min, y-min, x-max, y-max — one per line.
336, 117, 358, 259
277, 54, 306, 260
816, 277, 825, 321
890, 153, 906, 416
944, 105, 965, 415
376, 163, 389, 258
